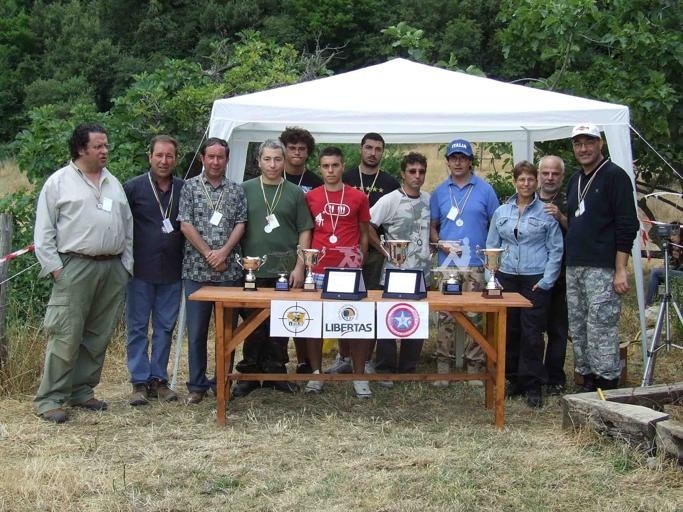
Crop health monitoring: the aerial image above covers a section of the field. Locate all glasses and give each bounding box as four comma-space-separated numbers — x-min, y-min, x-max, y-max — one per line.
574, 142, 595, 148
448, 155, 469, 161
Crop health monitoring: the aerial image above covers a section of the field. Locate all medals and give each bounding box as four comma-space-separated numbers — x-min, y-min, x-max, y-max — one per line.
161, 226, 168, 234
417, 238, 423, 247
264, 223, 273, 234
456, 218, 464, 226
329, 232, 338, 244
575, 208, 581, 217
95, 202, 103, 210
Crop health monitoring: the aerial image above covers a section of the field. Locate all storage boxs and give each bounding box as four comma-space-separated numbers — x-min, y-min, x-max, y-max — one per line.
567, 335, 631, 388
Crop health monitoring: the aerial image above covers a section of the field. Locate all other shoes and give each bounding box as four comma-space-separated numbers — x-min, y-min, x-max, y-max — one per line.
506, 374, 619, 409
232, 360, 261, 396
41, 408, 69, 424
71, 398, 107, 411
295, 363, 309, 386
185, 391, 205, 403
262, 364, 300, 394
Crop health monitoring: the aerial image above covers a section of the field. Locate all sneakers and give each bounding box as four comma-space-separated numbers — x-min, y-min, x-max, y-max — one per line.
149, 378, 178, 402
378, 379, 393, 388
365, 362, 375, 374
304, 370, 324, 395
324, 352, 353, 375
129, 383, 147, 406
353, 381, 371, 397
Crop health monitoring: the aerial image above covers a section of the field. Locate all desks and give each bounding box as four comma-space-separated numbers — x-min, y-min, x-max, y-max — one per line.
188, 285, 534, 428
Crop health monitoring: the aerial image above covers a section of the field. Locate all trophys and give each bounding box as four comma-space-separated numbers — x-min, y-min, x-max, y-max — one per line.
235, 253, 267, 291
379, 235, 416, 268
438, 241, 463, 295
476, 245, 509, 300
296, 244, 325, 292
276, 273, 289, 291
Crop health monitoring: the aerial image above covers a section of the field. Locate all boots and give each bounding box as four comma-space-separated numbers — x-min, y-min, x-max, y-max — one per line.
433, 364, 450, 387
467, 362, 483, 387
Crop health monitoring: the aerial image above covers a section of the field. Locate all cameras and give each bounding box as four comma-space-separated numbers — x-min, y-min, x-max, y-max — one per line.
649, 223, 672, 241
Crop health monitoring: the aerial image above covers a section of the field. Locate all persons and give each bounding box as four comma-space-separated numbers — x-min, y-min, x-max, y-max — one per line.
326, 133, 401, 373
567, 124, 639, 392
429, 140, 500, 388
176, 138, 249, 404
232, 140, 314, 397
279, 128, 324, 373
646, 222, 682, 307
485, 161, 563, 408
305, 147, 371, 397
34, 125, 134, 423
369, 153, 431, 387
536, 155, 568, 395
123, 136, 186, 404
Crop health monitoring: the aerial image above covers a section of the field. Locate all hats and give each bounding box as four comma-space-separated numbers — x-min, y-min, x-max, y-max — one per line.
571, 123, 601, 139
447, 140, 472, 156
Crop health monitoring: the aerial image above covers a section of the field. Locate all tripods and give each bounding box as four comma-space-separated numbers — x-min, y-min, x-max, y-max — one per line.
640, 241, 683, 387
